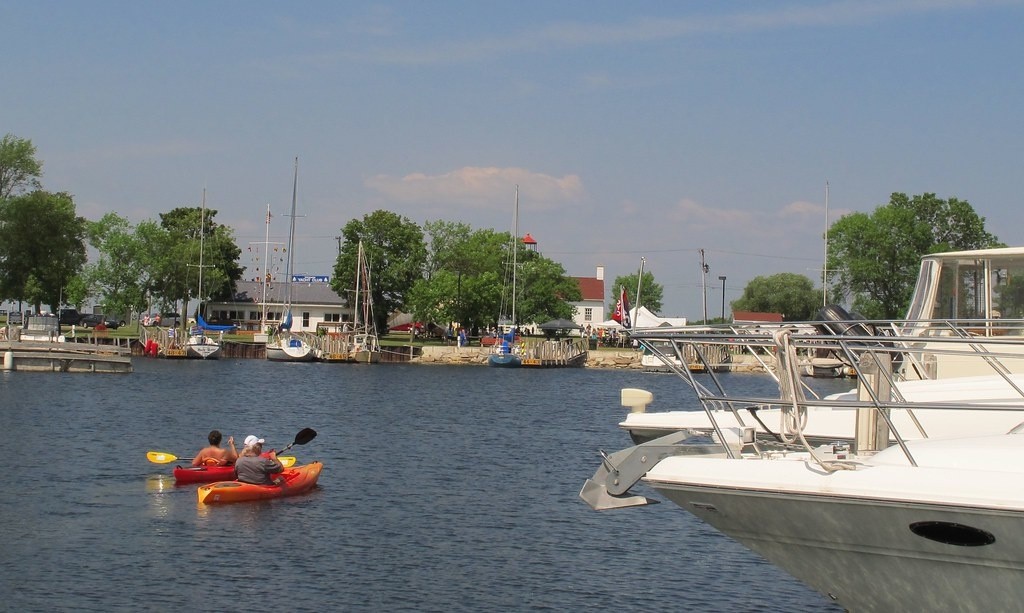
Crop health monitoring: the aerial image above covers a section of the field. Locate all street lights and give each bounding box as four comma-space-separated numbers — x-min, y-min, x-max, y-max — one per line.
719, 276, 726, 324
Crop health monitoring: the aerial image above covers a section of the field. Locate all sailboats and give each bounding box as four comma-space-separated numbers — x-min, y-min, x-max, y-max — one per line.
265, 156, 320, 360
183, 188, 221, 359
349, 239, 382, 362
489, 185, 523, 367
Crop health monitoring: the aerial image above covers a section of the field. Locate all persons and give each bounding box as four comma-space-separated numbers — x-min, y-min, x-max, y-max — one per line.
491, 326, 496, 338
447, 326, 467, 347
192, 430, 239, 466
235, 434, 283, 486
142, 313, 160, 326
579, 323, 616, 343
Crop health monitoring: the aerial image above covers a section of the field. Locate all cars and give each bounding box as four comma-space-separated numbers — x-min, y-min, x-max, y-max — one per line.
59, 309, 82, 325
79, 314, 119, 329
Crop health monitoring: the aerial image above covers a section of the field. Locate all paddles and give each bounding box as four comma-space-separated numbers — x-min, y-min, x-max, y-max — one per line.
268, 428, 317, 460
146, 452, 194, 464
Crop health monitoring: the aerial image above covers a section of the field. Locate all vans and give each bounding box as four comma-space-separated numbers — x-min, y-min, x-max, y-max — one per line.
144, 313, 181, 328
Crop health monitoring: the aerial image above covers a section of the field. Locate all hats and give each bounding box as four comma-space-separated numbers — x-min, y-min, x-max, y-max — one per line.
241, 435, 266, 445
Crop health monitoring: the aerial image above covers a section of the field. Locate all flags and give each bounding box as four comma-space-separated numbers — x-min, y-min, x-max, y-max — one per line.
612, 290, 632, 329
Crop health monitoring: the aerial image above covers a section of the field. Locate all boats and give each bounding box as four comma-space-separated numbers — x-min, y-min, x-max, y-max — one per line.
198, 462, 322, 506
580, 246, 1024, 613
173, 457, 296, 483
